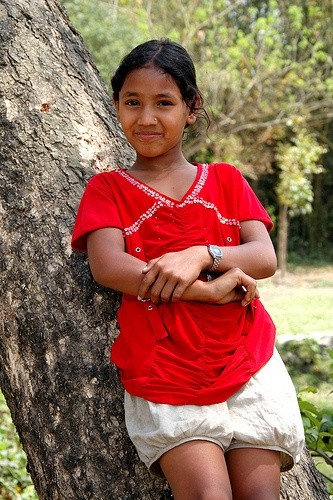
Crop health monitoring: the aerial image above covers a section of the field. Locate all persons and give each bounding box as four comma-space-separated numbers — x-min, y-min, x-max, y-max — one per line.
71, 40, 307, 500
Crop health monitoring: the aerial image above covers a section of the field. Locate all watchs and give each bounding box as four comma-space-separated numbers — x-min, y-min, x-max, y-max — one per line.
205, 246, 224, 274
